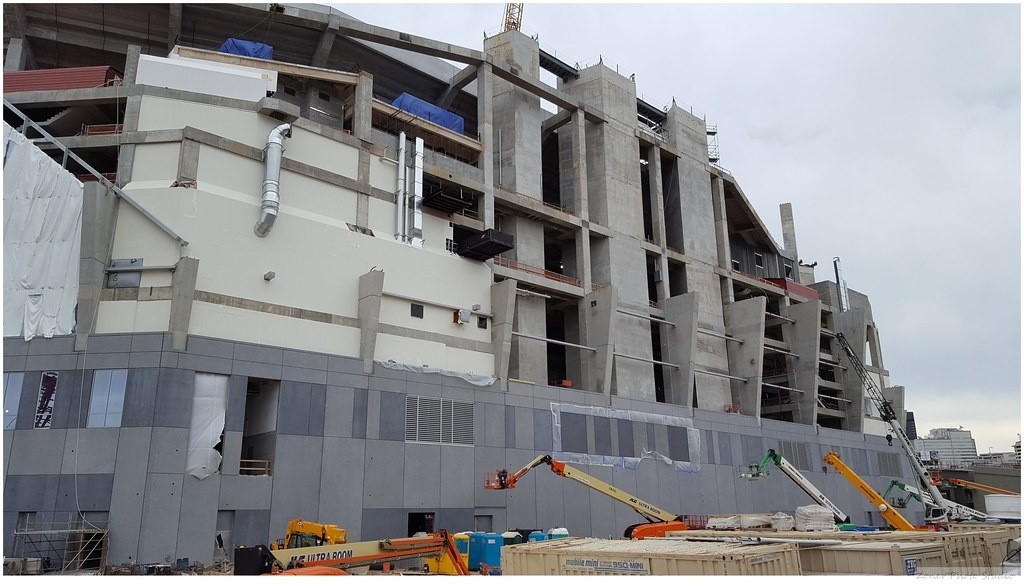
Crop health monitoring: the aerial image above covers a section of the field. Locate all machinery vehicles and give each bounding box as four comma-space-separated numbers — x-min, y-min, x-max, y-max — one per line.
268, 517, 349, 551
741, 448, 857, 531
821, 452, 936, 532
835, 330, 955, 524
263, 528, 471, 576
882, 478, 988, 525
938, 477, 1018, 495
482, 454, 688, 540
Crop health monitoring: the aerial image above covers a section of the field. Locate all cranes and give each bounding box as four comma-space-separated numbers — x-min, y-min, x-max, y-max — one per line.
503, 3, 524, 31
